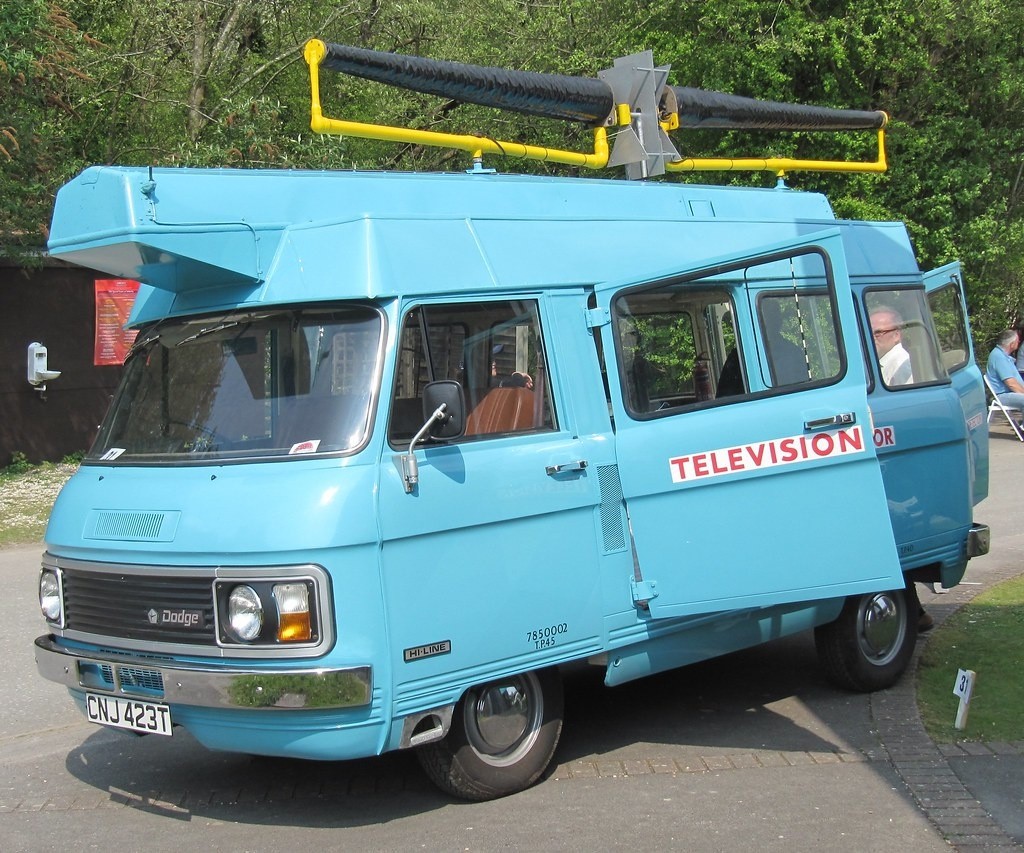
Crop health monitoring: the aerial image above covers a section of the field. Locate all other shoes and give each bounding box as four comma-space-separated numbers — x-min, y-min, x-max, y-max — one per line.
918, 611, 934, 632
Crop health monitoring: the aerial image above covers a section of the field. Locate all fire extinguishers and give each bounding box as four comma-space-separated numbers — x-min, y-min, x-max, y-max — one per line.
693, 352, 714, 401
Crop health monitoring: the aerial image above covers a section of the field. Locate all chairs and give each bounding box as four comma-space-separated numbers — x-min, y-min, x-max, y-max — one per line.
459, 383, 537, 437
983, 375, 1024, 442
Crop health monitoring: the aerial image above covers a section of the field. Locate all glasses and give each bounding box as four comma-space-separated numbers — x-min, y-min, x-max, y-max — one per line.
874, 327, 898, 339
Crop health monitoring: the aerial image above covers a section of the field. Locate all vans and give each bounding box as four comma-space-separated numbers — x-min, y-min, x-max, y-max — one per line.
36, 40, 993, 804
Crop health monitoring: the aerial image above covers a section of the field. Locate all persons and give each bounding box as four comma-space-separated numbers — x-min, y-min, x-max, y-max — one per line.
459, 344, 534, 388
1016, 341, 1024, 381
867, 308, 934, 634
985, 329, 1024, 433
713, 299, 810, 398
